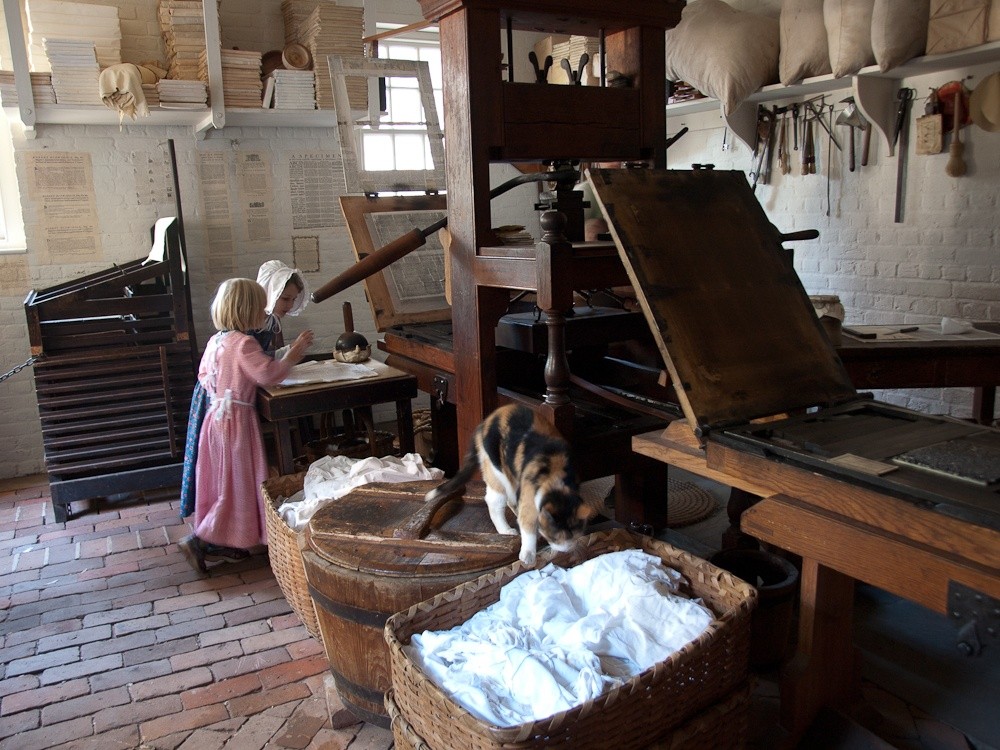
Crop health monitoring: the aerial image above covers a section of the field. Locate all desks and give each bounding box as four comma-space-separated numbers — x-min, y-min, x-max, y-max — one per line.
833, 321, 1000, 426
256, 355, 420, 477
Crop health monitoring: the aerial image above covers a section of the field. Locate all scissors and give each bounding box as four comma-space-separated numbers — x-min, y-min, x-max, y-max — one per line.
893, 87, 913, 146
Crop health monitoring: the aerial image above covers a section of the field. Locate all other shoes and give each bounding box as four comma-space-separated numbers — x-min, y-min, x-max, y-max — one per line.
206, 544, 244, 563
179, 535, 206, 575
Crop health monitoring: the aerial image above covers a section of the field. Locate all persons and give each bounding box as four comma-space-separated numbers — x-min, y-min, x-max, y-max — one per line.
177, 278, 304, 575
179, 260, 313, 561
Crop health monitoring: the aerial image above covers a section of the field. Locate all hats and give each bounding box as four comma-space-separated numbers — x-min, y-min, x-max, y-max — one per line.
256, 259, 309, 316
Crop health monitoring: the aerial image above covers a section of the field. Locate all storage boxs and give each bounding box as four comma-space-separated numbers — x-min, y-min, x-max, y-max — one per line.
929, 0, 988, 19
925, 6, 988, 57
987, 0, 1000, 43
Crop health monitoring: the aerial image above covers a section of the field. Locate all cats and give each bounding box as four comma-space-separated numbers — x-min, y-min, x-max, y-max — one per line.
424, 404, 593, 567
